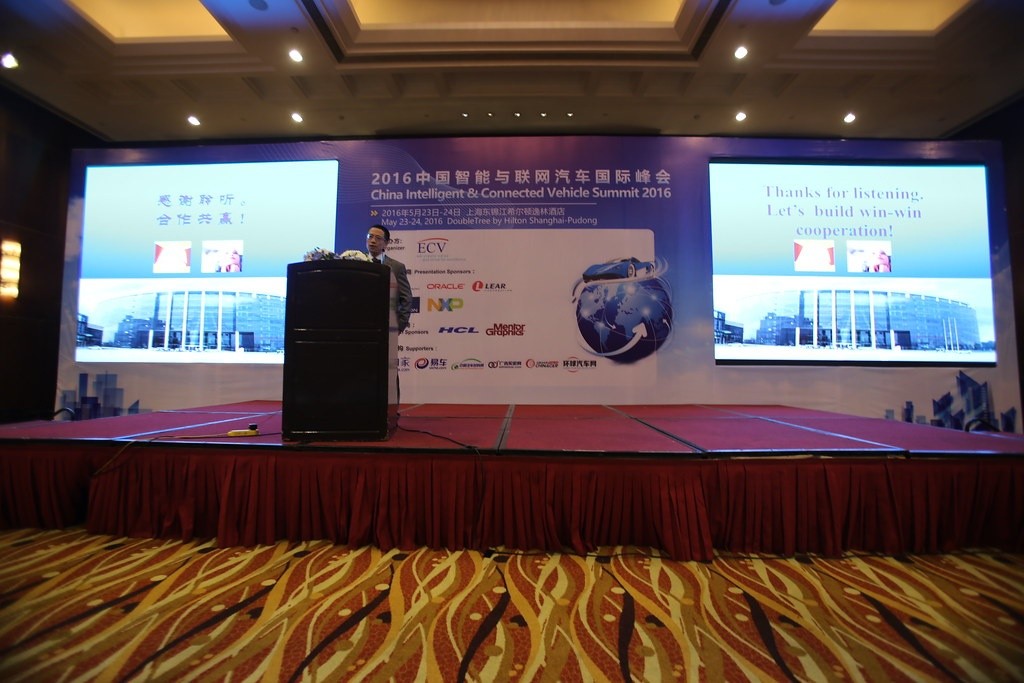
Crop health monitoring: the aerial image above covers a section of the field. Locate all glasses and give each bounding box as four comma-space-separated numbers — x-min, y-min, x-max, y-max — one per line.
367, 233, 385, 241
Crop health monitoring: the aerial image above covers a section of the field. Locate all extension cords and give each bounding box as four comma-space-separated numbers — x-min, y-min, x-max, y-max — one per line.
227, 429, 259, 436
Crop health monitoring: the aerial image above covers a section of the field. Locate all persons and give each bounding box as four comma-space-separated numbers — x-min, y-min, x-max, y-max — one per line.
366, 224, 414, 417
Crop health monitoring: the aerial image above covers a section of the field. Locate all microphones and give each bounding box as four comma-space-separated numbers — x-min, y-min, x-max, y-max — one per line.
382, 248, 385, 265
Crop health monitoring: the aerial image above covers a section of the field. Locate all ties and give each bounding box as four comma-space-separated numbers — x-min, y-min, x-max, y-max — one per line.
371, 257, 382, 264
